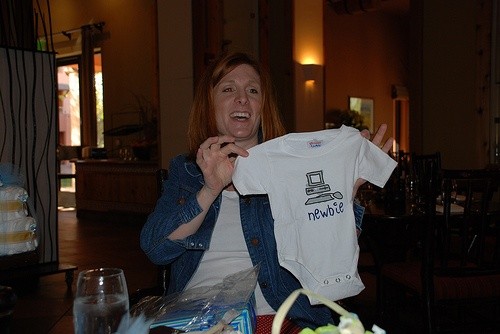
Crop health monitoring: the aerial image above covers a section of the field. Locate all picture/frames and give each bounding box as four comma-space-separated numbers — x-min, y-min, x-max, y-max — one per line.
348, 96, 374, 135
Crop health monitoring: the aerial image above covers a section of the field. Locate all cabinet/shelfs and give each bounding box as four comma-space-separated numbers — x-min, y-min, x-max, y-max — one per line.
75, 158, 160, 215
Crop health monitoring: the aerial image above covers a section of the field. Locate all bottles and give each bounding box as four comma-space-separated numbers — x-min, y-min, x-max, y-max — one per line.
437, 174, 474, 212
357, 173, 417, 215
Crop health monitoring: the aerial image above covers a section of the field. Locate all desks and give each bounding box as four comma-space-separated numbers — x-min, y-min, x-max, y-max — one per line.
360, 196, 465, 262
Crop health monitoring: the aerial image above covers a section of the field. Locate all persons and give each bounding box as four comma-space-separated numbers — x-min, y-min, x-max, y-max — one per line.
138, 50, 395, 334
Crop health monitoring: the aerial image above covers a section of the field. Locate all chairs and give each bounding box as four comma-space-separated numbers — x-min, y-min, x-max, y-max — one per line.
360, 150, 500, 334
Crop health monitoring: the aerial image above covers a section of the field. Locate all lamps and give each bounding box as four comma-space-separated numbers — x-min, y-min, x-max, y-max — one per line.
303, 64, 321, 80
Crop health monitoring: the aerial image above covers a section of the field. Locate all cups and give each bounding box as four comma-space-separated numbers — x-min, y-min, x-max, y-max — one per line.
119, 148, 127, 160
416, 192, 424, 215
471, 192, 481, 214
74, 268, 129, 334
